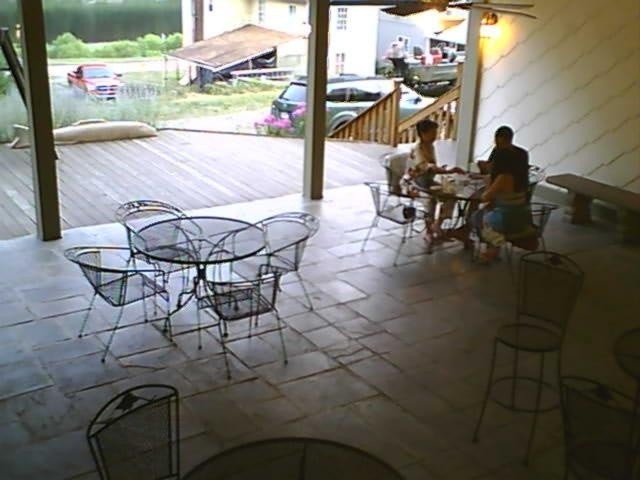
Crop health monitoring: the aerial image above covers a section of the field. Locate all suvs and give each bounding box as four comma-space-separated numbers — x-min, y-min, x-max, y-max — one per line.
270, 74, 435, 134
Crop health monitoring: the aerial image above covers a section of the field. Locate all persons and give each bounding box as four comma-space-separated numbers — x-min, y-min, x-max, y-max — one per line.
400, 119, 464, 242
448, 127, 528, 242
414, 41, 457, 64
478, 150, 532, 260
382, 37, 409, 62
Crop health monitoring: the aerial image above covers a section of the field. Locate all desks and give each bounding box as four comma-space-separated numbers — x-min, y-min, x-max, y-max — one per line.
182, 437, 405, 480
134, 217, 266, 338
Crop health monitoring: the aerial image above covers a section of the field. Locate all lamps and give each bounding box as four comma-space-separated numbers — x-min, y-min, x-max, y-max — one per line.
410, 17, 465, 35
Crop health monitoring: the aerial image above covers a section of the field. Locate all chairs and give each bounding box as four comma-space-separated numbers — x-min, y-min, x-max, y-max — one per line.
528, 164, 546, 199
558, 376, 639, 480
229, 212, 321, 311
194, 264, 289, 379
360, 182, 432, 267
472, 202, 559, 285
85, 383, 179, 480
114, 199, 202, 317
63, 246, 173, 363
472, 250, 585, 466
378, 152, 427, 238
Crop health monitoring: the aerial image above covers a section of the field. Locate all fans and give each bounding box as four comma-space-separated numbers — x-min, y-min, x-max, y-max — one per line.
329, 0, 538, 19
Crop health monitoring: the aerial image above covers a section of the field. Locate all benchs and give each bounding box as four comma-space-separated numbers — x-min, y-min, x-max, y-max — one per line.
546, 173, 639, 238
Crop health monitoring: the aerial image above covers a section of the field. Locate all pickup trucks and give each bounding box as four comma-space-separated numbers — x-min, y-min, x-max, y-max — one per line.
67, 64, 124, 99
